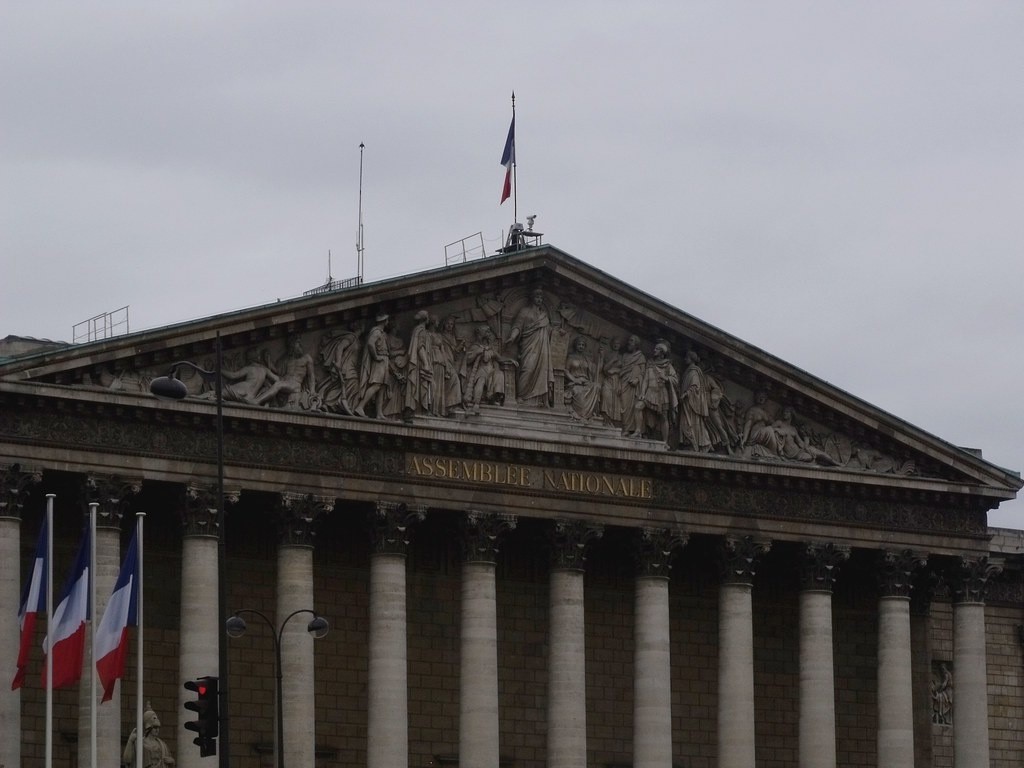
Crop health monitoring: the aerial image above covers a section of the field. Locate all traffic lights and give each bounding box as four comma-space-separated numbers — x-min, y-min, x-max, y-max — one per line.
182, 674, 219, 757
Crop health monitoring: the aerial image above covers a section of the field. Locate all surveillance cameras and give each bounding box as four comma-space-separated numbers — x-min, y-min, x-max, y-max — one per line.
527, 214, 536, 219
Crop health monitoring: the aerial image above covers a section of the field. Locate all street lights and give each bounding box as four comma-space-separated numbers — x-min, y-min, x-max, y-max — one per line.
224, 608, 331, 768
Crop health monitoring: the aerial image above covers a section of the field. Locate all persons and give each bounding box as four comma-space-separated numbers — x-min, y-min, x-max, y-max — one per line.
97, 285, 915, 475
937, 663, 952, 705
122, 699, 177, 768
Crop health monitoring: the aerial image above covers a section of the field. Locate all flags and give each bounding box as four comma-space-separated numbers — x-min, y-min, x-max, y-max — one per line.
41, 523, 91, 692
95, 527, 140, 704
500, 110, 516, 201
11, 514, 48, 691
